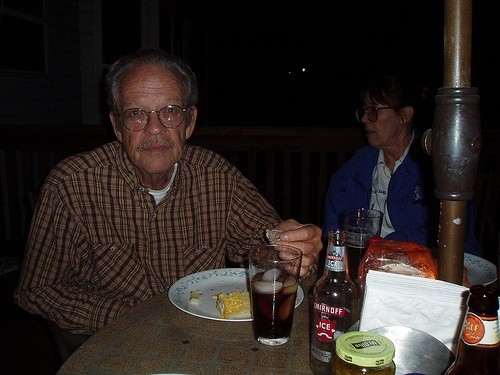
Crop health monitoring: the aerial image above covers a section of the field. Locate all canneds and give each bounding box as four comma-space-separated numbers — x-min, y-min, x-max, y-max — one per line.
330, 331, 396, 375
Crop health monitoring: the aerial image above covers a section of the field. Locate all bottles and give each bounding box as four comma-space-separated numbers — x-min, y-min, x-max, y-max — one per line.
309, 228, 359, 374
443, 284, 500, 375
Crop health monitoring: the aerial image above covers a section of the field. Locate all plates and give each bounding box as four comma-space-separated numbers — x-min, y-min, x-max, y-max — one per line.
461, 253, 498, 288
168, 268, 304, 322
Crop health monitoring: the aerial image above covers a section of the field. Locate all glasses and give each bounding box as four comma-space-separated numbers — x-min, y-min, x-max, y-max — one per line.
115, 104, 189, 133
363, 105, 392, 122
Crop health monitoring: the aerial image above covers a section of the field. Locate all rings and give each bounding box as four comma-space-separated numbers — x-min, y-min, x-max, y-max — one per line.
308, 264, 319, 274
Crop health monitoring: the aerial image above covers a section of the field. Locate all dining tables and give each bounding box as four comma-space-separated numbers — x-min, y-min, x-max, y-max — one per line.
55, 263, 321, 375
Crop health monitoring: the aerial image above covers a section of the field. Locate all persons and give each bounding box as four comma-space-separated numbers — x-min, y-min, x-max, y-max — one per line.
13, 49, 323, 362
322, 75, 484, 268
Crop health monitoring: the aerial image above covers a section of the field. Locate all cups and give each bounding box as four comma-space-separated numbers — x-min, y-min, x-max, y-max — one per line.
249, 245, 303, 347
341, 208, 383, 285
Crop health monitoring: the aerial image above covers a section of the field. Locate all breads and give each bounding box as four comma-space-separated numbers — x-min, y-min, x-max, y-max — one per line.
216, 292, 252, 318
266, 229, 284, 244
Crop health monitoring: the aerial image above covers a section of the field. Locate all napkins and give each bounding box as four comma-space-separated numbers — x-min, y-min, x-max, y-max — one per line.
359, 269, 470, 353
